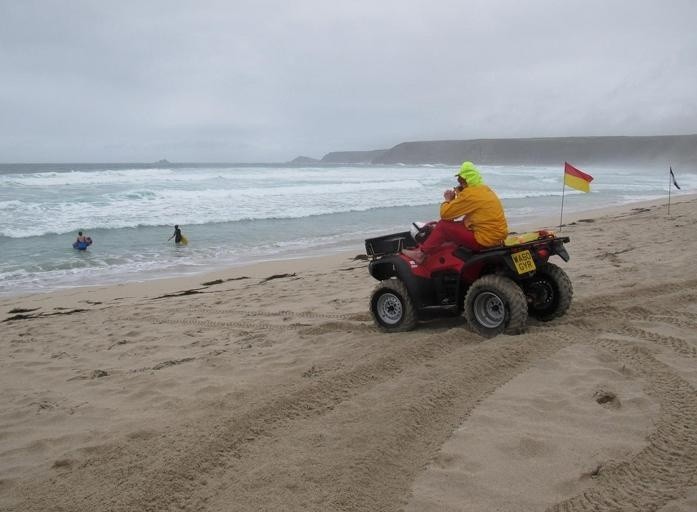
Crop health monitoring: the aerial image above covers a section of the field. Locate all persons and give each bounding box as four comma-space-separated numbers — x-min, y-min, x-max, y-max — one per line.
168, 225, 181, 244
75, 232, 91, 253
398, 162, 508, 264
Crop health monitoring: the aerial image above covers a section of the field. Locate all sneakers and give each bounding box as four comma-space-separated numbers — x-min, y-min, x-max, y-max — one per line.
401, 248, 427, 265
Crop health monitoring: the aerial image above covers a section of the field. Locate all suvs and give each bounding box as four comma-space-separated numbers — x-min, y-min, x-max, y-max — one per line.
364, 221, 573, 339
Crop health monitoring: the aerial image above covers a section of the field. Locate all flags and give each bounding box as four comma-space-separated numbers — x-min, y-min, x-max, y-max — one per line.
670, 165, 680, 190
564, 162, 593, 197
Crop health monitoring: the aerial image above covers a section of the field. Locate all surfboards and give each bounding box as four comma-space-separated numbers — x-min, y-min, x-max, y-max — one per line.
72, 237, 92, 248
181, 235, 187, 246
